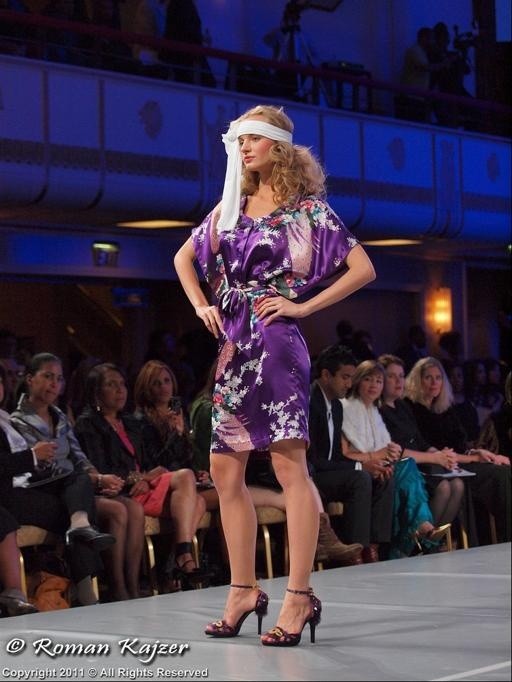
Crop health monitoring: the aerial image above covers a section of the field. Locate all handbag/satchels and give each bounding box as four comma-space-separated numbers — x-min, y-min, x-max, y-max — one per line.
415, 462, 452, 474
24, 570, 72, 613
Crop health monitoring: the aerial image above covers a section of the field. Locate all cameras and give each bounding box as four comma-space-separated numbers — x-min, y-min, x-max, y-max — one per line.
169, 396, 183, 414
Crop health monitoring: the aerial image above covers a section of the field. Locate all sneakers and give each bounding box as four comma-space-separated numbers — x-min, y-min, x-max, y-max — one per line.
340, 542, 381, 566
1, 586, 38, 615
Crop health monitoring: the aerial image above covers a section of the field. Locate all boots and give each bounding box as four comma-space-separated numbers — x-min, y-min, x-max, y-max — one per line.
317, 512, 364, 562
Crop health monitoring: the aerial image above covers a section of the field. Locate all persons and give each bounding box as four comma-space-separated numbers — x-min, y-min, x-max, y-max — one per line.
0, 1, 512, 132
0, 317, 511, 622
171, 103, 381, 648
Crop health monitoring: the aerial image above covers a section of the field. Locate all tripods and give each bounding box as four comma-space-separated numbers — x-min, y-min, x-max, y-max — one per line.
276, 30, 335, 105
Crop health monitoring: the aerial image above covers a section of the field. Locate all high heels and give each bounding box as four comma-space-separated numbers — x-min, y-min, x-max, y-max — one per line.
203, 582, 270, 640
63, 522, 116, 554
168, 543, 215, 584
410, 521, 453, 550
261, 587, 323, 649
